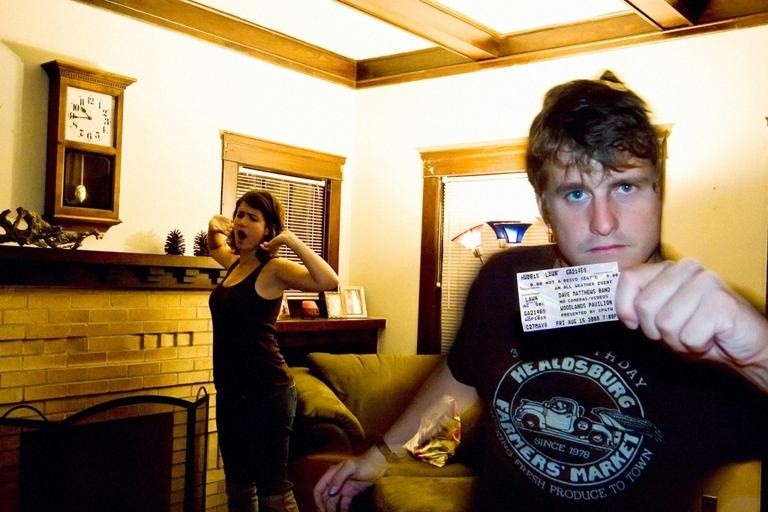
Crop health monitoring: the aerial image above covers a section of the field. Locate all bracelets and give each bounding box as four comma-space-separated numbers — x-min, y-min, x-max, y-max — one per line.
374, 432, 402, 465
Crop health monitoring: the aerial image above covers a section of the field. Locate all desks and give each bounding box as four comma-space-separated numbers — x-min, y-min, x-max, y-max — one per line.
271, 318, 386, 354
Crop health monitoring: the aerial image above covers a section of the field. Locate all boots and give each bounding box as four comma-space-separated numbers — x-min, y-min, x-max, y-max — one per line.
225, 485, 299, 512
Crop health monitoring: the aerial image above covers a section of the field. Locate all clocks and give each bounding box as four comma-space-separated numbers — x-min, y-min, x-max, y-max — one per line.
38, 60, 138, 224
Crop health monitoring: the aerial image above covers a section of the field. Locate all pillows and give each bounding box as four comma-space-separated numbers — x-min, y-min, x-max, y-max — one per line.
308, 351, 481, 443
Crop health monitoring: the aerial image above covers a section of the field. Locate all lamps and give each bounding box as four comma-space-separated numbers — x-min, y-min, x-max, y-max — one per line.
449, 222, 531, 266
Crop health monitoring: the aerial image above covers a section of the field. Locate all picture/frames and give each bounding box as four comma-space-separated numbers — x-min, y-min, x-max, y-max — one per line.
324, 290, 348, 319
338, 285, 368, 319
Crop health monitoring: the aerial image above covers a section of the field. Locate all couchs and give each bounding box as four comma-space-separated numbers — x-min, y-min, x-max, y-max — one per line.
287, 366, 762, 512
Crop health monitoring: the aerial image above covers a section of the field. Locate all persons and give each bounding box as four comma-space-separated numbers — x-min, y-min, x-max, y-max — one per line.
311, 77, 768, 510
206, 188, 341, 511
